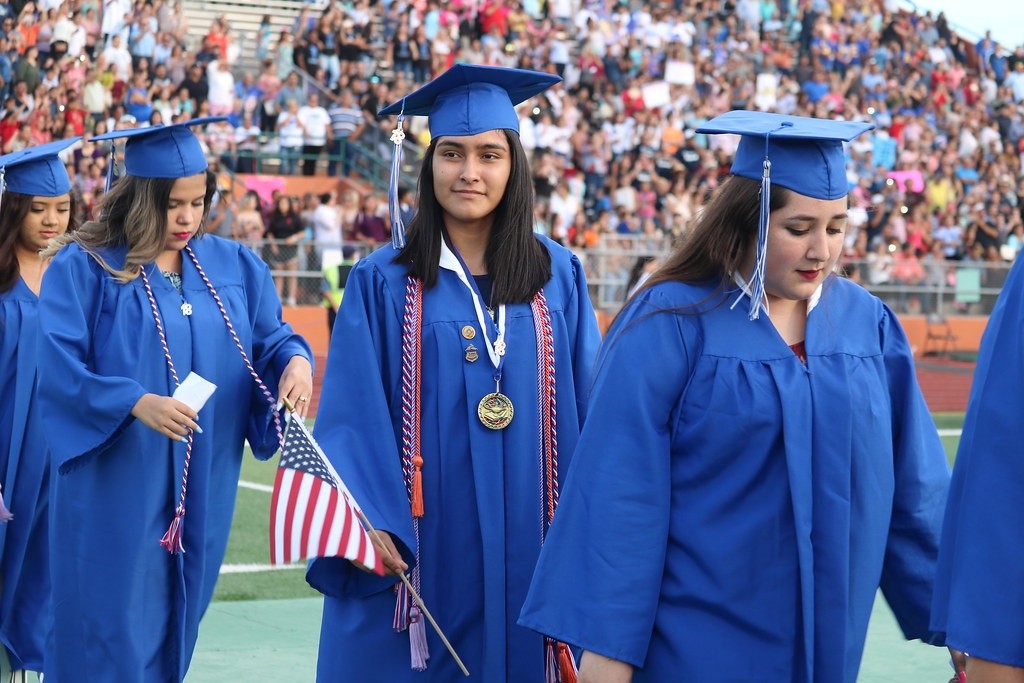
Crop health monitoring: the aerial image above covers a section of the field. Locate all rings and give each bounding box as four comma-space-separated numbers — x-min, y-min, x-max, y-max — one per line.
298, 396, 307, 402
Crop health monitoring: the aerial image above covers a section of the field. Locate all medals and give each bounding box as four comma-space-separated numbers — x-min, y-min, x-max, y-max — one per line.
477, 393, 514, 430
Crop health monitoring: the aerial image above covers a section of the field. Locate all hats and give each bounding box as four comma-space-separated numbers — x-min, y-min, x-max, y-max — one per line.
376, 63, 564, 250
0, 136, 83, 197
694, 111, 876, 322
86, 116, 231, 195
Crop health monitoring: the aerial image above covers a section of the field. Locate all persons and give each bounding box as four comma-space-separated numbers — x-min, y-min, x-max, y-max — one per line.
928, 244, 1024, 683
0, 119, 315, 683
305, 63, 603, 683
0, 1, 1024, 317
515, 110, 967, 683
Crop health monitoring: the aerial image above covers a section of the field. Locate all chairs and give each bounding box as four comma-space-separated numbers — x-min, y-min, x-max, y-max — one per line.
922, 313, 959, 355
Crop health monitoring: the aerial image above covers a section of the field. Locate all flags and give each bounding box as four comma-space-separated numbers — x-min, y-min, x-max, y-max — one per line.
270, 414, 386, 577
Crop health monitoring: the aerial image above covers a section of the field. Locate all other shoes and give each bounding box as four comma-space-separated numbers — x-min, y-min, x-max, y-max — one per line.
278, 296, 283, 304
286, 297, 297, 306
